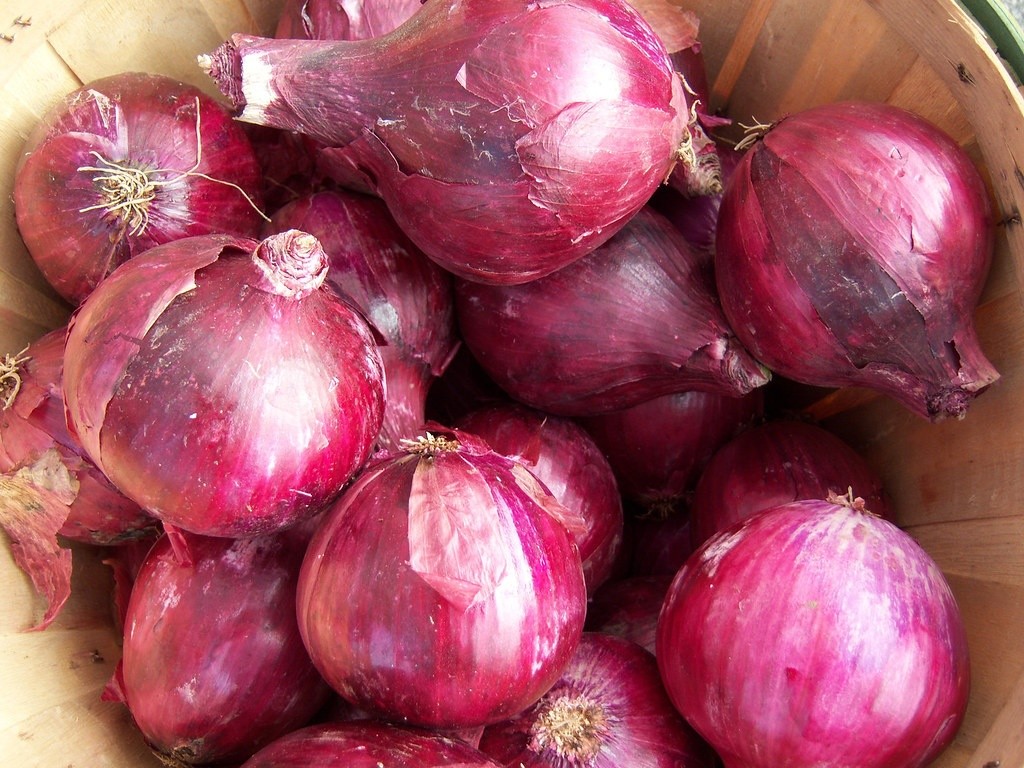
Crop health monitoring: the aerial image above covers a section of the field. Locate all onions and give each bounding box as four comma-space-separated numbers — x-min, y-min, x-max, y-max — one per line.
0, 0, 1000, 768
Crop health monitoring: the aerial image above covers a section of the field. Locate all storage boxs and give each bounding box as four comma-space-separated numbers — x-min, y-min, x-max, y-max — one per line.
0, 0, 1024, 768
